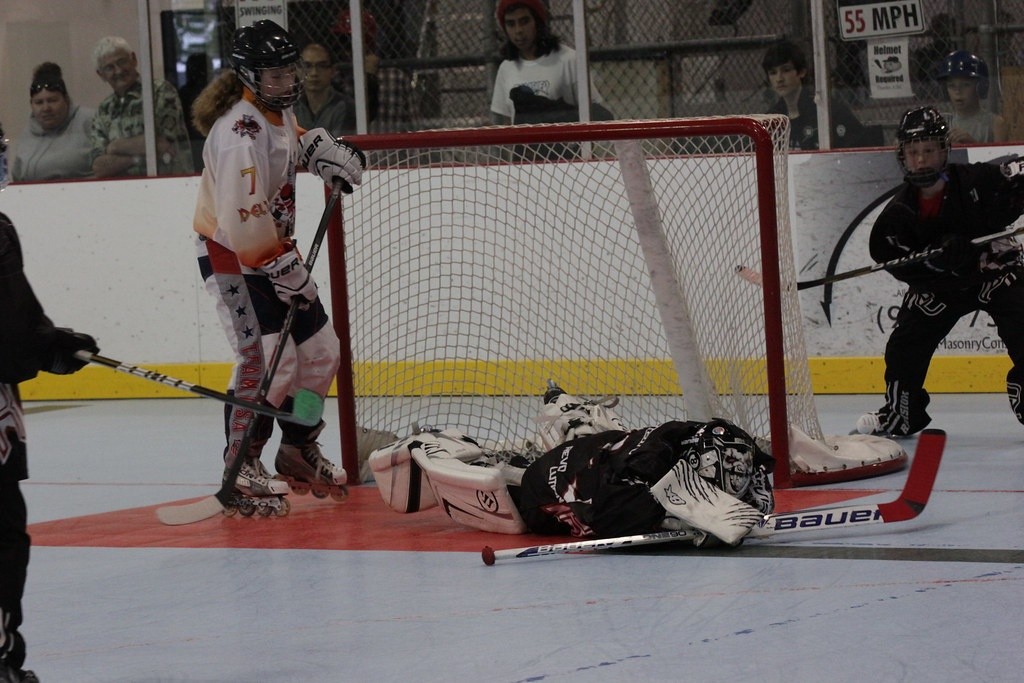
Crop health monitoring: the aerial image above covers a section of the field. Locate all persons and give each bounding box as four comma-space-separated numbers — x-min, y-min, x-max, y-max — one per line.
762, 41, 860, 146
8, 61, 94, 180
90, 35, 195, 179
935, 50, 1008, 145
191, 19, 362, 518
0, 122, 101, 683
369, 378, 775, 543
330, 9, 415, 166
854, 106, 1024, 435
492, 0, 604, 160
295, 41, 355, 135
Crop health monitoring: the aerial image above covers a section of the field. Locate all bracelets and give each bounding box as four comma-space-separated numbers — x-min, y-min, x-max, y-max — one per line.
134, 157, 139, 164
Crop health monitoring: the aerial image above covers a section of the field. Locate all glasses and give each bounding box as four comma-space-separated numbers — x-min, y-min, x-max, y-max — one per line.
29, 82, 66, 99
300, 62, 332, 71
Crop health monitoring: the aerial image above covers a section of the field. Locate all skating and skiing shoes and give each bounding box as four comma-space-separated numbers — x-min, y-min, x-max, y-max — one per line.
849, 411, 912, 440
215, 457, 291, 517
273, 417, 349, 501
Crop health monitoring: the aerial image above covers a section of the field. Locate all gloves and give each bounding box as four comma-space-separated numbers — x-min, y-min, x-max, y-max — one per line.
257, 239, 317, 310
932, 238, 988, 269
39, 326, 101, 376
295, 127, 367, 195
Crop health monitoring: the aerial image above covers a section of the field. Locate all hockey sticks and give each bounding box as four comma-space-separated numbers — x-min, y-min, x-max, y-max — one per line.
154, 173, 342, 525
734, 225, 1024, 293
70, 348, 324, 430
482, 428, 946, 567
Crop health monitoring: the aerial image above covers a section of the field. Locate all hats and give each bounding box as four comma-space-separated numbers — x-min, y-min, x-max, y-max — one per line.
495, 0, 549, 34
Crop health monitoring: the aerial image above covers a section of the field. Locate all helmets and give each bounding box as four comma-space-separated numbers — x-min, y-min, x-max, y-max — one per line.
935, 50, 989, 102
681, 418, 756, 500
896, 106, 952, 184
225, 18, 304, 113
